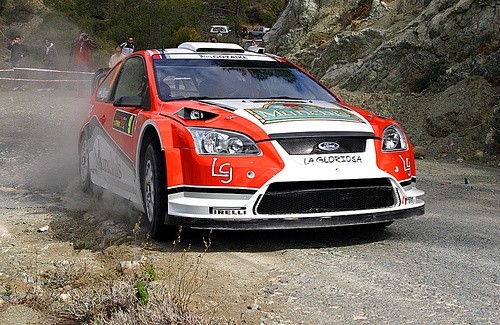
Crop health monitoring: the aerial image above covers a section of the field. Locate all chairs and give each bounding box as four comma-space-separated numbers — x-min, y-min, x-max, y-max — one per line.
199, 79, 253, 97
144, 82, 171, 107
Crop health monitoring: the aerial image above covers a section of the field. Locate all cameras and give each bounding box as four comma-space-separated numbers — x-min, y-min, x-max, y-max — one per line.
81, 34, 88, 39
13, 40, 18, 43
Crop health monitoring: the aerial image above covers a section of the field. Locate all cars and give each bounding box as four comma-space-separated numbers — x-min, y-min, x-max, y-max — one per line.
210, 25, 231, 33
249, 26, 271, 36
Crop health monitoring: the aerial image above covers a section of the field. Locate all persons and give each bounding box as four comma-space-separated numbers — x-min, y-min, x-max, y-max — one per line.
120, 36, 135, 54
6, 36, 27, 91
71, 34, 94, 97
210, 26, 263, 46
109, 45, 126, 68
41, 39, 60, 90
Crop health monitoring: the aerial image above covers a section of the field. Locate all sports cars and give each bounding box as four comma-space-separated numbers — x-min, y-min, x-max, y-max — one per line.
77, 41, 427, 240
